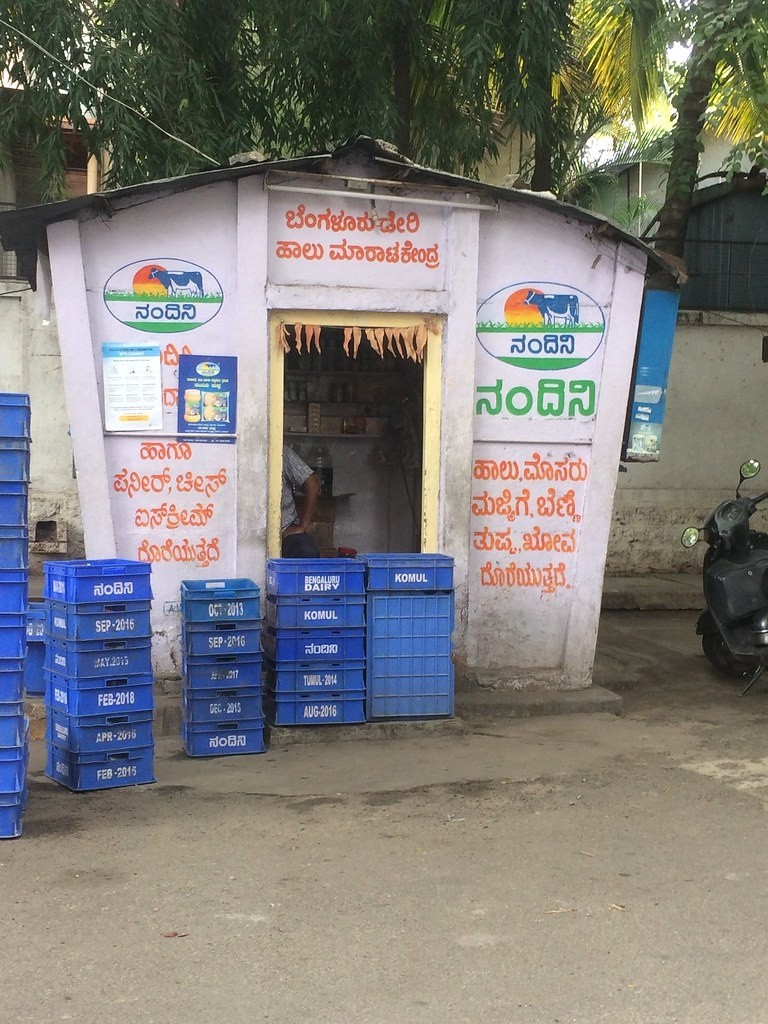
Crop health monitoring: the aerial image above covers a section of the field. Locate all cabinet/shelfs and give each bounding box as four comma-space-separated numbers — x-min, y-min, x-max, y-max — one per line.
283, 346, 388, 438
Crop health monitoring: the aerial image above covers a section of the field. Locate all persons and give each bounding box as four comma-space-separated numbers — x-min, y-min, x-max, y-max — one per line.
282, 444, 321, 559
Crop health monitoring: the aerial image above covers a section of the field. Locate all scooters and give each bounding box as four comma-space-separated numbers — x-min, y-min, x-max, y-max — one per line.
681, 459, 768, 695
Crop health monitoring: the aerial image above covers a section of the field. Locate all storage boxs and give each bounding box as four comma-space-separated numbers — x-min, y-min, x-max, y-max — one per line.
178, 579, 268, 757
0, 393, 162, 840
290, 402, 388, 434
262, 548, 456, 727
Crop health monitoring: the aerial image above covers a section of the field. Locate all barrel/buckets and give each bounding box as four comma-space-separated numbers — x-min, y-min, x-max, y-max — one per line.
307, 441, 334, 499
